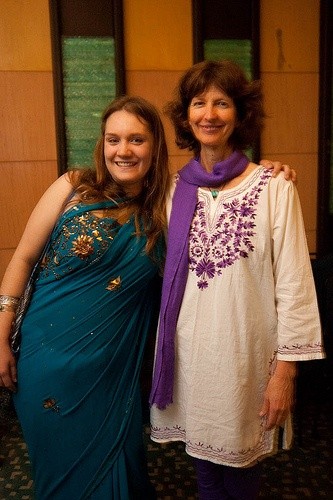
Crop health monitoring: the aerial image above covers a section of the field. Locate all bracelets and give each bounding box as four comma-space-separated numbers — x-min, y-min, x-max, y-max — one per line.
0, 294, 21, 314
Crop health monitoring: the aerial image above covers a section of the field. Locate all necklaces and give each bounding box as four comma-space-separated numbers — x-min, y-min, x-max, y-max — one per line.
207, 179, 226, 198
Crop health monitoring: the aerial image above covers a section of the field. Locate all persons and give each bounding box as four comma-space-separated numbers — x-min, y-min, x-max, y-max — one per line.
149, 59, 325, 499
1, 94, 299, 500
